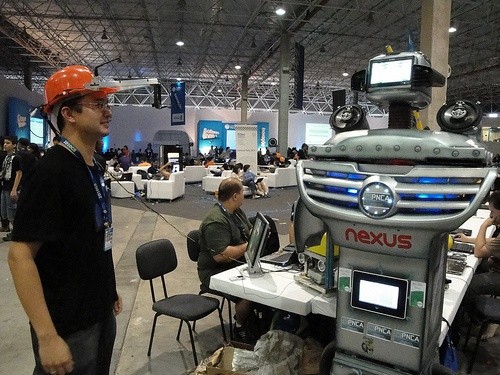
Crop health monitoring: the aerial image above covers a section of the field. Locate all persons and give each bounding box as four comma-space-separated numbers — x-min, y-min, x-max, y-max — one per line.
96, 139, 312, 200
8, 66, 125, 375
196, 175, 279, 344
489, 153, 500, 189
0, 132, 59, 242
455, 189, 500, 342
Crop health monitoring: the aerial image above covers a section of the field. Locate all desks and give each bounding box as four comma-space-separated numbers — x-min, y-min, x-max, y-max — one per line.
258, 164, 275, 169
208, 206, 497, 375
128, 166, 150, 173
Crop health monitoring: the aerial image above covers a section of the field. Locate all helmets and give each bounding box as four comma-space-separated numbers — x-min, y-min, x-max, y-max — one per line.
45, 65, 119, 114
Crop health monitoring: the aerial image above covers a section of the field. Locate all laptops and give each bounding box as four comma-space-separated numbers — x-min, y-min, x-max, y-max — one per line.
450, 242, 475, 254
261, 249, 298, 265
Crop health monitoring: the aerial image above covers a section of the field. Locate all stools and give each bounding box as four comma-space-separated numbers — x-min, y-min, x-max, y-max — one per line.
110, 181, 135, 199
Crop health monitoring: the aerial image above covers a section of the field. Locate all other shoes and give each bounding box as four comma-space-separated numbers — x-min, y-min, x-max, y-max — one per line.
231, 322, 252, 344
254, 190, 263, 194
3, 232, 13, 242
0, 226, 11, 232
261, 193, 271, 198
253, 195, 260, 199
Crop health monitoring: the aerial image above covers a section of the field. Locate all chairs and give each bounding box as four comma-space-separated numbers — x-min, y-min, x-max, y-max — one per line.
184, 166, 206, 185
146, 170, 186, 205
187, 229, 244, 342
132, 174, 145, 192
202, 175, 229, 196
222, 169, 253, 199
135, 237, 228, 367
261, 167, 298, 189
249, 215, 281, 259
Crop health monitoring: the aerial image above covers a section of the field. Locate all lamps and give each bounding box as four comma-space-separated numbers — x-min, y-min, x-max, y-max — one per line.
217, 86, 222, 92
319, 43, 326, 53
251, 35, 257, 49
275, 4, 286, 16
176, 58, 183, 67
101, 27, 108, 39
366, 10, 374, 23
19, 26, 30, 41
448, 21, 460, 33
342, 68, 350, 77
234, 58, 242, 70
313, 80, 322, 91
225, 75, 229, 81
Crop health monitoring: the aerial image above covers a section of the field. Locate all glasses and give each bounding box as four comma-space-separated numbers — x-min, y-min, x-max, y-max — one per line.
76, 102, 113, 112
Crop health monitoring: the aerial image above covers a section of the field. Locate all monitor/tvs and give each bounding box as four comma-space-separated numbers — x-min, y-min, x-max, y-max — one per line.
240, 210, 271, 279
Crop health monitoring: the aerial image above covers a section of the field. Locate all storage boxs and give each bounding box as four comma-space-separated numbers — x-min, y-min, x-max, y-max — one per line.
205, 340, 258, 375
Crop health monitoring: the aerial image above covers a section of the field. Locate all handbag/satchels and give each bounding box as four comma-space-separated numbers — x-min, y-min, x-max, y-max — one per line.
441, 333, 459, 371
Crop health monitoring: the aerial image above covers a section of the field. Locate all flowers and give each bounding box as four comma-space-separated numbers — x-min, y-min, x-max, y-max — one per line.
273, 152, 286, 166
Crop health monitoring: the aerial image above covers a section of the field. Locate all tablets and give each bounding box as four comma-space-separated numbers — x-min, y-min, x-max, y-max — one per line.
367, 54, 417, 90
351, 269, 409, 320
